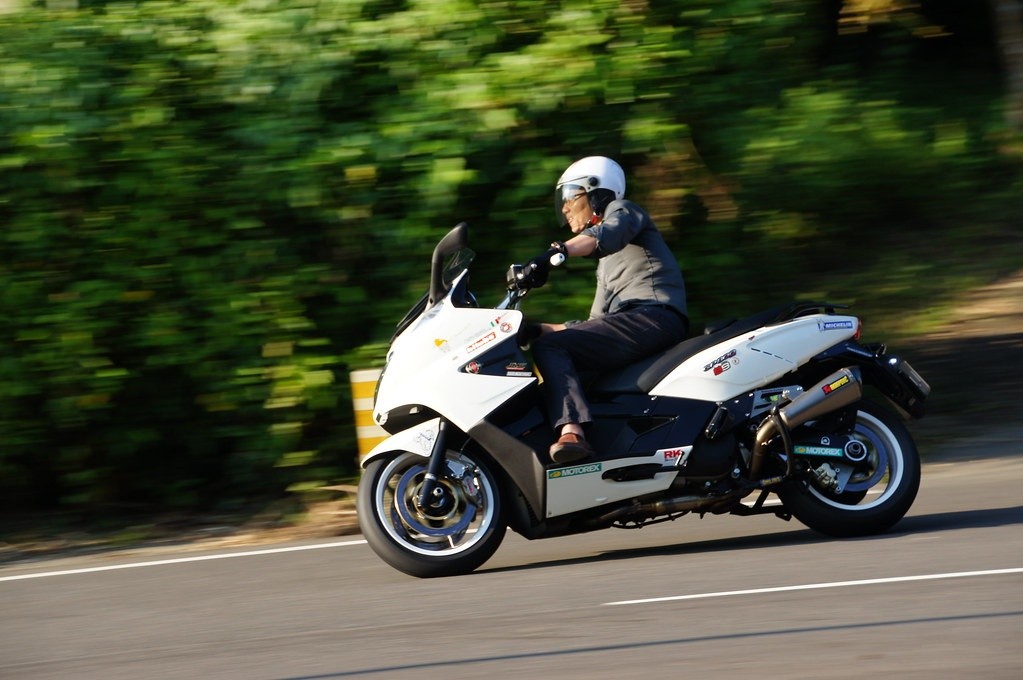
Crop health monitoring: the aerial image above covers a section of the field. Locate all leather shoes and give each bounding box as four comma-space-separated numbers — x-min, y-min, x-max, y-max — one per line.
550, 432, 596, 464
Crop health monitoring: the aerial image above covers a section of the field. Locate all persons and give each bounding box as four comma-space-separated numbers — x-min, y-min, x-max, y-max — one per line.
504, 156, 690, 466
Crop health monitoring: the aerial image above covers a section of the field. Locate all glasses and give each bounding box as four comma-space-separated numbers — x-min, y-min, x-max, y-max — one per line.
561, 192, 586, 207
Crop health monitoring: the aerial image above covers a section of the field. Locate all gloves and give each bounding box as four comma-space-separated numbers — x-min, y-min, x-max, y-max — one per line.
518, 323, 541, 346
517, 247, 559, 289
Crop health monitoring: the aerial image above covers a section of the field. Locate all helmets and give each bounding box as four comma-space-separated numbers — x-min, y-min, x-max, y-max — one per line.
556, 156, 626, 200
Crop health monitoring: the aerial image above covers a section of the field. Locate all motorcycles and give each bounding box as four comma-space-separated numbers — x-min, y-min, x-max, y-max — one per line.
355, 221, 946, 579
